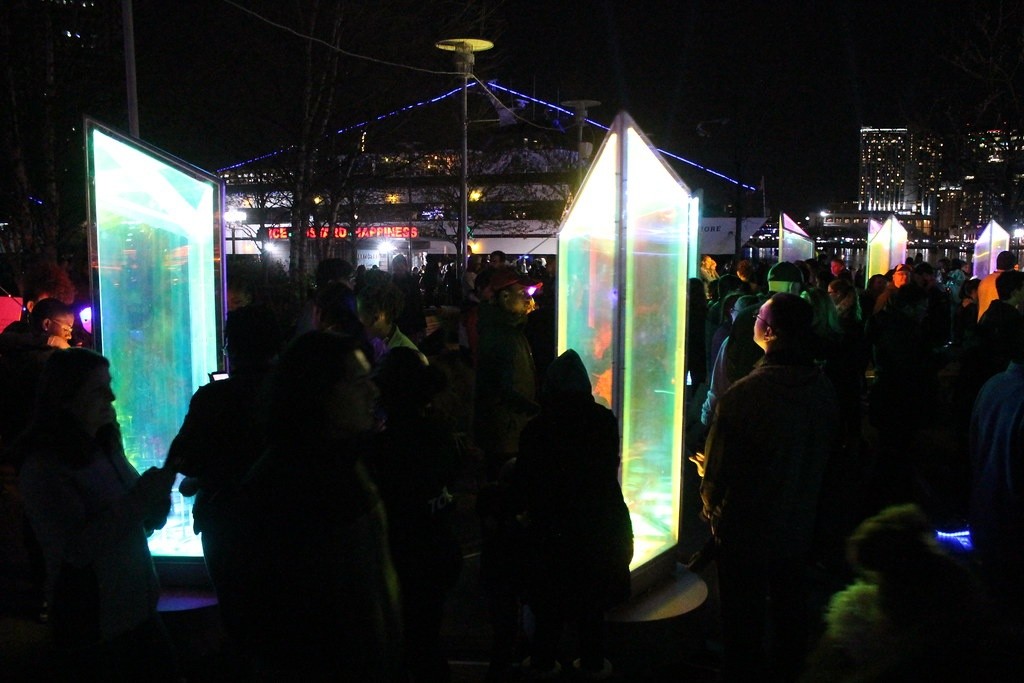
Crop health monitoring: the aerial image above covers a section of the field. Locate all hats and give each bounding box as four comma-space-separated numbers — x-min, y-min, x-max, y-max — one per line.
490, 269, 543, 290
767, 261, 805, 285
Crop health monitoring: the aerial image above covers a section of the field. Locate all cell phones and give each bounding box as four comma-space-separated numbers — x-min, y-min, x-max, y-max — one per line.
211, 371, 229, 381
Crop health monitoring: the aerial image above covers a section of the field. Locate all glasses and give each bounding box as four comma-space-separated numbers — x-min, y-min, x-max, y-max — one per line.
50, 319, 73, 334
752, 308, 776, 333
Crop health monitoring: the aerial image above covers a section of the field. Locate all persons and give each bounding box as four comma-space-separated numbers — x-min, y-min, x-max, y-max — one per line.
0, 250, 1024, 683
